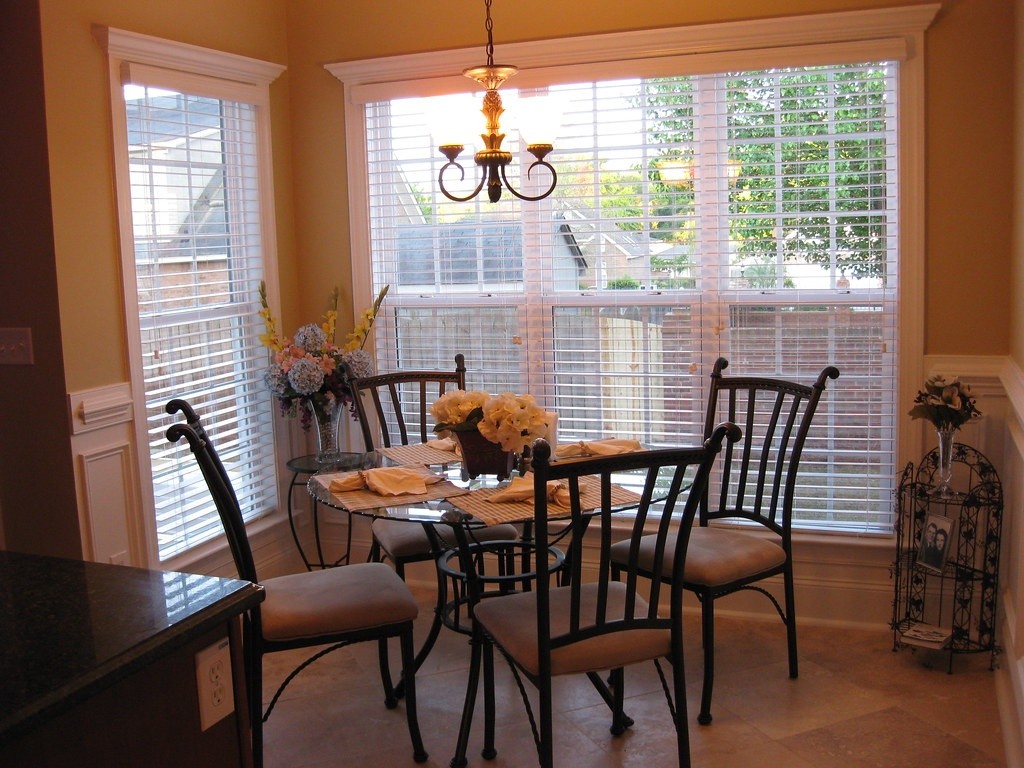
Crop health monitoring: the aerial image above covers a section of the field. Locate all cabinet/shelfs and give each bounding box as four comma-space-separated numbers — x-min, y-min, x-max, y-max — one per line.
0, 551, 266, 768
889, 442, 1005, 675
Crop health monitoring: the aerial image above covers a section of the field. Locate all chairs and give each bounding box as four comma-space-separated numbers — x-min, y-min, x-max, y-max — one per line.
607, 358, 843, 727
448, 420, 743, 767
345, 352, 519, 595
163, 398, 430, 768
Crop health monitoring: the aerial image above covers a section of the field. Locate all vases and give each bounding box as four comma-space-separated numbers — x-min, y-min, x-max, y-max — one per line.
455, 430, 513, 482
311, 398, 345, 464
926, 428, 960, 499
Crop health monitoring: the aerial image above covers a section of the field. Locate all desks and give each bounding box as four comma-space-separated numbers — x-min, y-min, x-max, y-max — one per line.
306, 449, 699, 768
285, 451, 381, 572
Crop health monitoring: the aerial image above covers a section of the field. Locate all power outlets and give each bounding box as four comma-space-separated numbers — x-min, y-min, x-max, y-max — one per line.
109, 550, 128, 566
194, 636, 237, 732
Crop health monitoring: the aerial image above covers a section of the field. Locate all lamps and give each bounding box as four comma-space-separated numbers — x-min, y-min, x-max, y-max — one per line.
424, 1, 557, 204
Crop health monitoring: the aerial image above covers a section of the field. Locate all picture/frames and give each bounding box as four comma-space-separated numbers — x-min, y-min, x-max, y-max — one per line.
915, 510, 956, 575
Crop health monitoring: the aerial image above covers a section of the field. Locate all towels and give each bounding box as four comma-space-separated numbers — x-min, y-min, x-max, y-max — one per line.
328, 463, 449, 497
483, 470, 590, 507
557, 437, 640, 457
427, 436, 463, 457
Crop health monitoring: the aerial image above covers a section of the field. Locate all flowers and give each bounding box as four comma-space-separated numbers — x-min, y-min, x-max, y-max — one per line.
256, 280, 390, 447
907, 373, 985, 468
427, 387, 549, 456
